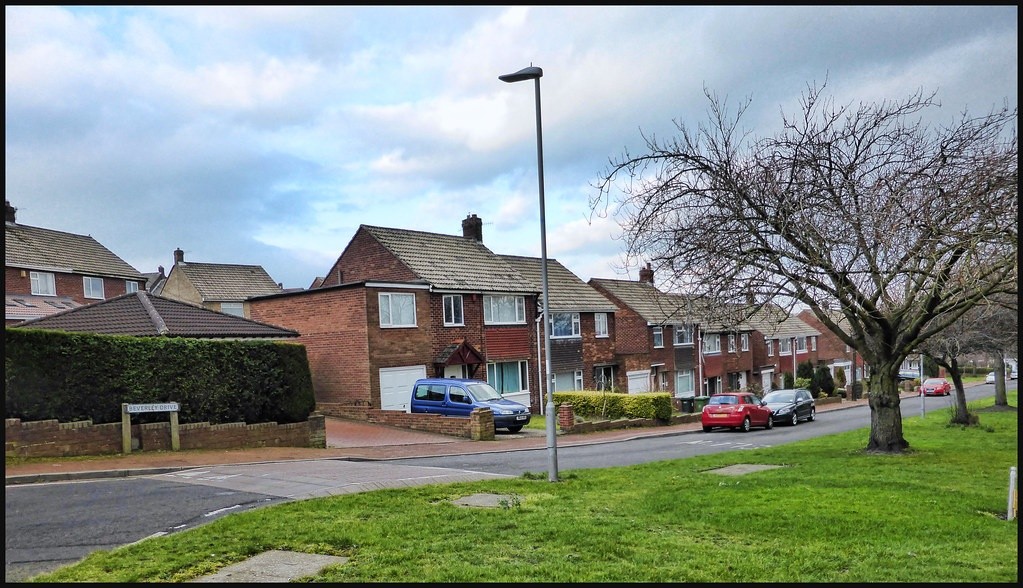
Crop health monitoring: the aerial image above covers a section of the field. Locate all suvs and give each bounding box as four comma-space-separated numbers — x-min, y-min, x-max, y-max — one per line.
410, 376, 531, 434
762, 388, 816, 425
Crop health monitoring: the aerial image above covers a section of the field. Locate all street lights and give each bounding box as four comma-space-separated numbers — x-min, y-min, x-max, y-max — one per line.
499, 66, 560, 484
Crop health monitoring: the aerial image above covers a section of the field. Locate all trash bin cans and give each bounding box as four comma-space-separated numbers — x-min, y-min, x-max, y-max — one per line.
680, 395, 694, 412
693, 396, 710, 420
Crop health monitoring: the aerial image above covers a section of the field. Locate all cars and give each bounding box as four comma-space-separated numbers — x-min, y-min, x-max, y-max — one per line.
918, 377, 951, 396
700, 391, 776, 433
986, 371, 995, 384
